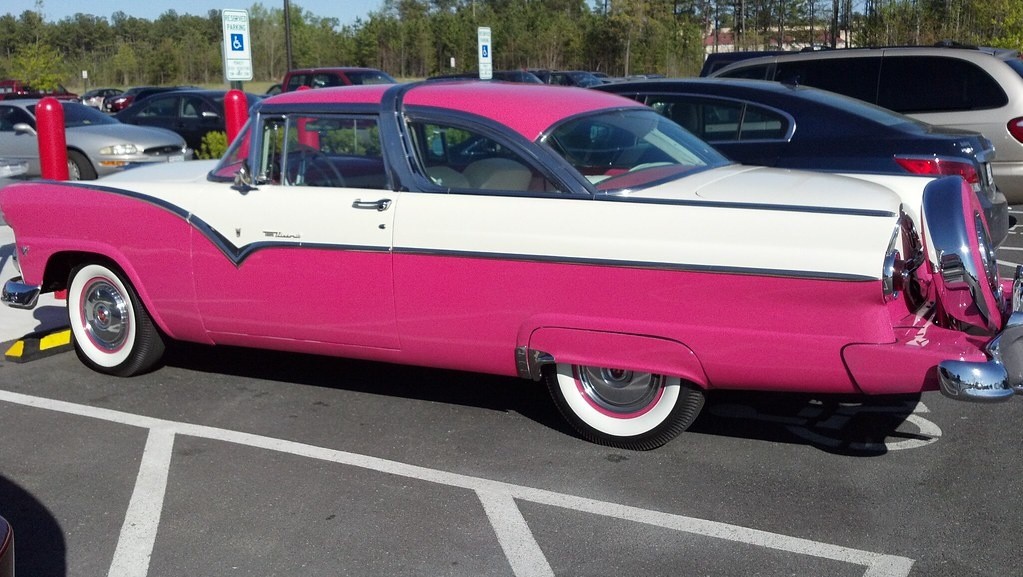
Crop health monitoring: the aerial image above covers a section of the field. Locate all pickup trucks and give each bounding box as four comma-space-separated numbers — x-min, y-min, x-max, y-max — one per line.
4, 82, 80, 103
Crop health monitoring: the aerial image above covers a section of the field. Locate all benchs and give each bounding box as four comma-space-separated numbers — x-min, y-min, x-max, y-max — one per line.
292, 160, 387, 191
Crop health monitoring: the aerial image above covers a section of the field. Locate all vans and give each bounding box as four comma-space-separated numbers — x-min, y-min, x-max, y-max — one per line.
282, 68, 399, 128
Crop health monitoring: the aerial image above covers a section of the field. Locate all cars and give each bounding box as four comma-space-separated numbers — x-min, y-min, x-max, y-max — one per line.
435, 76, 1018, 259
419, 70, 614, 91
0, 81, 1023, 451
0, 79, 205, 117
113, 89, 291, 161
0, 99, 196, 190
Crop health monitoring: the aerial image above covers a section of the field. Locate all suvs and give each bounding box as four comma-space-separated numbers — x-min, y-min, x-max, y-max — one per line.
623, 40, 1023, 211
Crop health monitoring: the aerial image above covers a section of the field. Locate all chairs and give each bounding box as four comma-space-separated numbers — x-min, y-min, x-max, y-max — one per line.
428, 157, 534, 190
